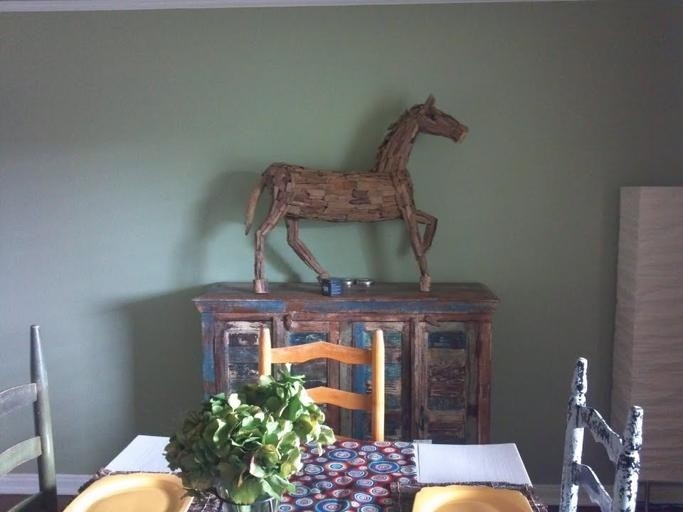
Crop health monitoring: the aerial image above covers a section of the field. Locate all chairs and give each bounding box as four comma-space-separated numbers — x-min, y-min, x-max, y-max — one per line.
557, 355, 644, 511
255, 325, 390, 444
0, 324, 61, 512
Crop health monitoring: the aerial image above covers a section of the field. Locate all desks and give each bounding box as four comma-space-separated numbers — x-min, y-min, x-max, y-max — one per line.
62, 433, 548, 512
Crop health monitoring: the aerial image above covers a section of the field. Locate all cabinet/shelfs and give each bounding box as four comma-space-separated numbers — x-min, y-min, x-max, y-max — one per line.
189, 280, 502, 445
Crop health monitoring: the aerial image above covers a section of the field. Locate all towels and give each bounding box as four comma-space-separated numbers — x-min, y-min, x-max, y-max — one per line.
105, 434, 182, 473
411, 442, 532, 487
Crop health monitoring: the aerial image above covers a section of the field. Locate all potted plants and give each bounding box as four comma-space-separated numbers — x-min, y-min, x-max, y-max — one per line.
160, 369, 336, 512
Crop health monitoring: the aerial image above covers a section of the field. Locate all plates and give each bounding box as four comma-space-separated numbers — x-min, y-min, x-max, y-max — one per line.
64, 472, 194, 512
410, 485, 532, 511
